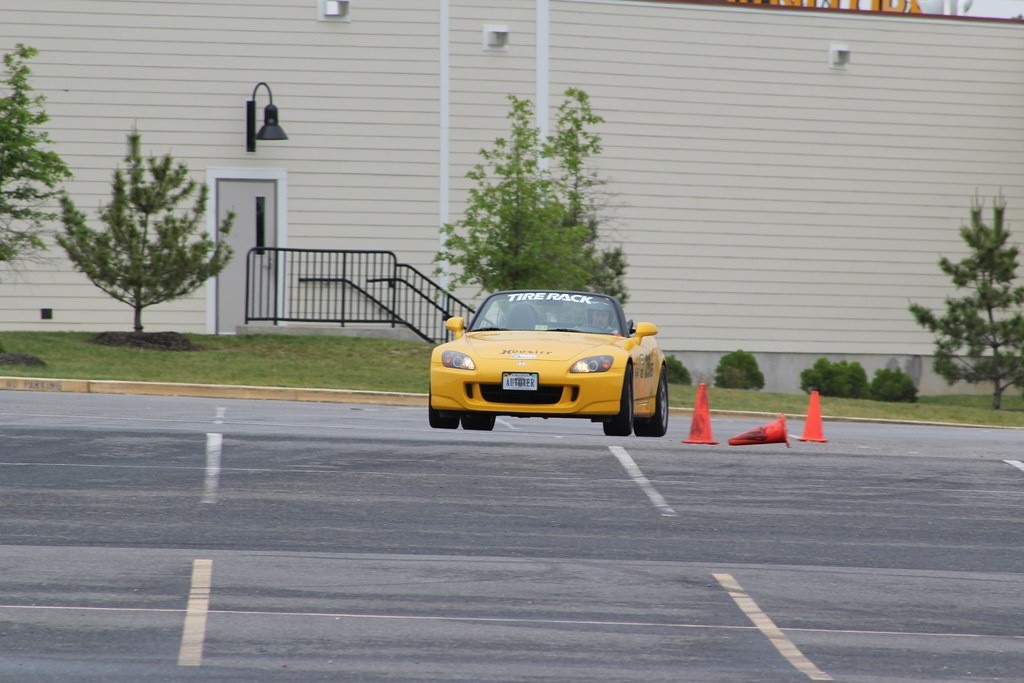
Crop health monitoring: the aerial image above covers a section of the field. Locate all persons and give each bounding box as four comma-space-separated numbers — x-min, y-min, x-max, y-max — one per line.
585, 301, 619, 333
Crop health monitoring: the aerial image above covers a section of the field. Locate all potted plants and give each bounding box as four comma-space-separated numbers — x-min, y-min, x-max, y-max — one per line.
713, 349, 766, 391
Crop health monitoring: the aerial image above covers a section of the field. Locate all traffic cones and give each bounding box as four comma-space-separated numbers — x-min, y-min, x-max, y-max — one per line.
681, 384, 721, 445
798, 390, 829, 443
727, 410, 791, 450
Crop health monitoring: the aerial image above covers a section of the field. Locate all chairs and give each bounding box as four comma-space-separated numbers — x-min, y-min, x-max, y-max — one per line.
499, 301, 545, 330
577, 303, 615, 332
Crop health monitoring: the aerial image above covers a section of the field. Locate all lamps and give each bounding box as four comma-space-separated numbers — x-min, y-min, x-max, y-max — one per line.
246, 82, 290, 152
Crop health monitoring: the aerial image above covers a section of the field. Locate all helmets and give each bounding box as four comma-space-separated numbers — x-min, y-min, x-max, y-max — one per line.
585, 299, 614, 328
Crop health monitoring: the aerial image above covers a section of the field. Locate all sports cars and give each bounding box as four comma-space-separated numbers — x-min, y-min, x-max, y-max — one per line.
428, 288, 671, 439
